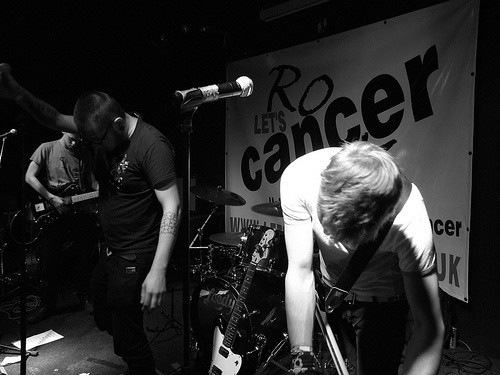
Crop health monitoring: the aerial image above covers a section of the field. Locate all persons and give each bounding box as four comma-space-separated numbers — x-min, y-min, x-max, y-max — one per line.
280, 140, 444, 375
25, 132, 99, 314
0, 63, 182, 375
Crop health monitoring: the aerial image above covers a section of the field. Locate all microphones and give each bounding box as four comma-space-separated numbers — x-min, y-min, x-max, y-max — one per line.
0, 127, 19, 139
174, 75, 254, 103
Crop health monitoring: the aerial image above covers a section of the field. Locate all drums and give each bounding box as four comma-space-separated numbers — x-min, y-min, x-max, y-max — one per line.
190, 273, 284, 359
239, 224, 287, 273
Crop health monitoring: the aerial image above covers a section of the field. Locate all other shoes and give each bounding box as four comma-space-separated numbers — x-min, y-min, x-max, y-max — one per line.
84, 299, 93, 314
27, 307, 56, 323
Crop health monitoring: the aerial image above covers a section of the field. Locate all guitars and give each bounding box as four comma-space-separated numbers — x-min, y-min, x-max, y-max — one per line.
313, 260, 349, 375
208, 228, 275, 375
31, 184, 99, 231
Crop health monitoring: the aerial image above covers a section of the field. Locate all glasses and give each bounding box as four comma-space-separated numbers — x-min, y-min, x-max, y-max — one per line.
78, 117, 117, 146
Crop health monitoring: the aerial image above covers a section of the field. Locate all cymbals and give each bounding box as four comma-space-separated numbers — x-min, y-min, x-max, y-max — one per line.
210, 233, 243, 246
190, 185, 246, 206
251, 202, 282, 217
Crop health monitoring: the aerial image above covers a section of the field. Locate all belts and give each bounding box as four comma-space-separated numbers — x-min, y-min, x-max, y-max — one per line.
330, 289, 408, 305
